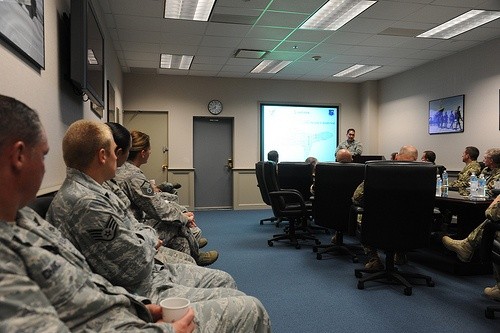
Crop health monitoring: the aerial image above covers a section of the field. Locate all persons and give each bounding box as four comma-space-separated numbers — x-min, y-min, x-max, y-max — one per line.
46, 120, 246, 307
0, 94, 272, 333
104, 121, 219, 267
437, 105, 463, 130
268, 128, 500, 300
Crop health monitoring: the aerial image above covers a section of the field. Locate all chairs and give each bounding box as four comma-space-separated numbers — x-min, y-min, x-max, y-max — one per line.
311, 161, 365, 263
254, 159, 288, 228
351, 160, 441, 298
261, 160, 321, 250
275, 160, 330, 235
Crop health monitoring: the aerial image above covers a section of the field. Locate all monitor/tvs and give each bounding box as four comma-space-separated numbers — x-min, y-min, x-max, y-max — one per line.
69, 0, 106, 108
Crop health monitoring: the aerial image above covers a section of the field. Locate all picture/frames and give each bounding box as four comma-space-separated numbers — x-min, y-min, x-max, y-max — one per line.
427, 93, 466, 136
0, 0, 47, 71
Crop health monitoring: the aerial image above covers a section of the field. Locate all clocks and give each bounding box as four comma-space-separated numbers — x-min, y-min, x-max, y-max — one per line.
208, 99, 224, 116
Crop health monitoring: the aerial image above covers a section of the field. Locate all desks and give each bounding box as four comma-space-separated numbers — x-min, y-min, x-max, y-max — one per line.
434, 185, 496, 276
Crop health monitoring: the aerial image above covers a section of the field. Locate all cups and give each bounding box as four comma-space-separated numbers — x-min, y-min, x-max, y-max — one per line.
160, 297, 190, 323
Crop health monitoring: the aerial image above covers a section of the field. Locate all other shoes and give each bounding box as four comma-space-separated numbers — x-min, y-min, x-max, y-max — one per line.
364, 259, 378, 270
332, 235, 336, 242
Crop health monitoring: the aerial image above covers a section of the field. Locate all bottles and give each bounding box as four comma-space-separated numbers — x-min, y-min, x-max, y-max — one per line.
470, 172, 478, 195
442, 170, 448, 186
435, 174, 442, 188
478, 174, 486, 195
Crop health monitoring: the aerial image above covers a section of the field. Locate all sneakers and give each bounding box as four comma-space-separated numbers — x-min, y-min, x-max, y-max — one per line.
195, 251, 218, 266
442, 236, 475, 262
484, 283, 500, 298
198, 237, 207, 248
